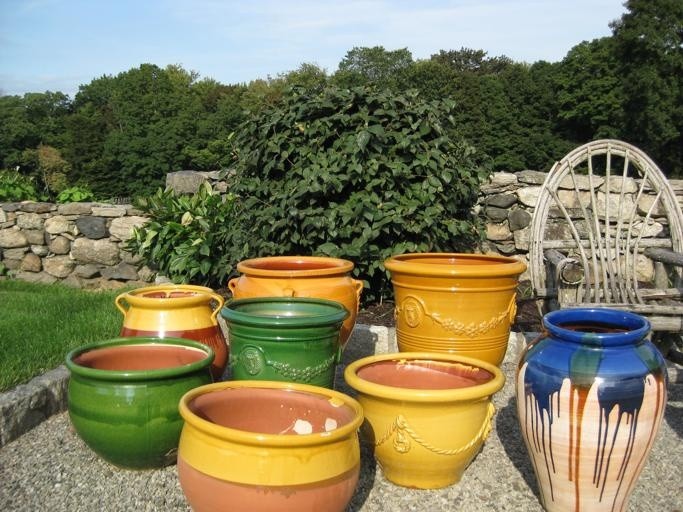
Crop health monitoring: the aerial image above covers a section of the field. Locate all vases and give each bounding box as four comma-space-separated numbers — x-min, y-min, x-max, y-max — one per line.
515, 307, 669, 512
221, 296, 349, 391
229, 255, 365, 353
344, 350, 505, 491
384, 251, 528, 372
65, 337, 215, 471
116, 284, 229, 379
177, 381, 364, 512
